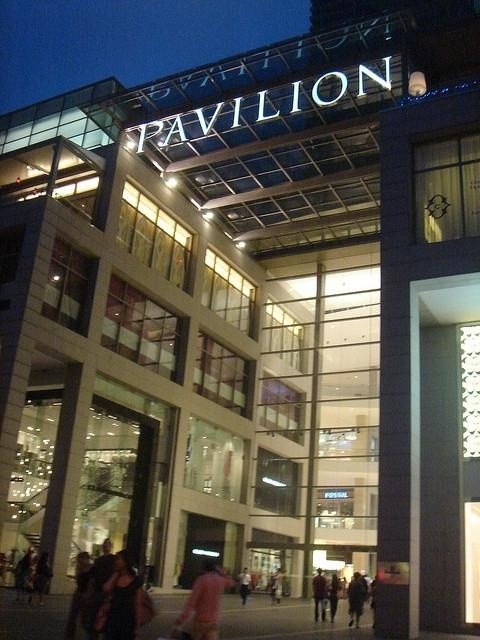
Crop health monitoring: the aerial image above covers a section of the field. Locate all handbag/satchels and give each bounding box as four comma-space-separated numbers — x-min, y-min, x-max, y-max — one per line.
93, 600, 111, 629
139, 589, 157, 624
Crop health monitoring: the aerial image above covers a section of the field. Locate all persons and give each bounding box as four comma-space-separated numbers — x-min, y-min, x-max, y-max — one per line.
100, 550, 144, 640
0, 543, 54, 610
236, 567, 252, 606
173, 558, 238, 640
87, 538, 118, 633
309, 564, 377, 630
62, 552, 101, 640
270, 567, 284, 607
